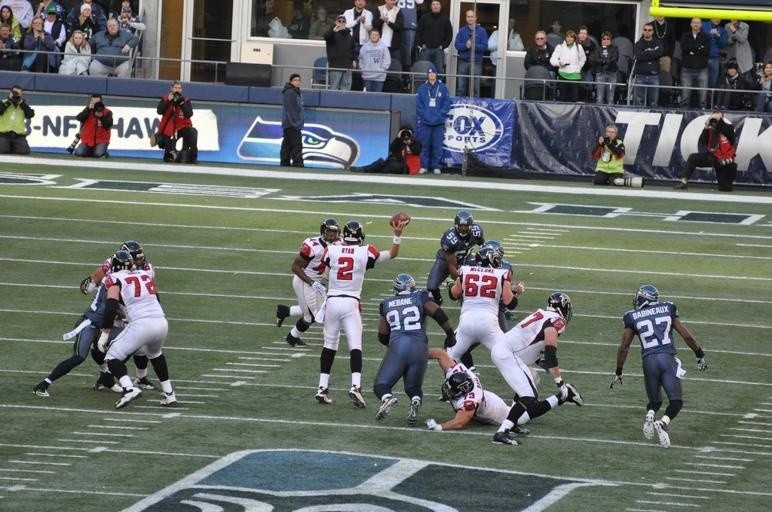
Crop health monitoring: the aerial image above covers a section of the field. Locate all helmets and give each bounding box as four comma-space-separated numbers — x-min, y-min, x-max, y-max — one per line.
478, 240, 504, 259
111, 250, 134, 272
392, 273, 416, 296
320, 217, 341, 244
120, 240, 145, 267
454, 210, 473, 237
440, 372, 474, 402
633, 284, 660, 311
475, 247, 502, 268
548, 292, 573, 324
343, 220, 365, 247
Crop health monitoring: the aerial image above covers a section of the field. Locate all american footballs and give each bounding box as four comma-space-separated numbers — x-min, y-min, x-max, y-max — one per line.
389, 212, 411, 227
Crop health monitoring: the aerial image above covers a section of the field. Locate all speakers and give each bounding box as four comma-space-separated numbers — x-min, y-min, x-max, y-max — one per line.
224, 62, 271, 87
709, 118, 717, 127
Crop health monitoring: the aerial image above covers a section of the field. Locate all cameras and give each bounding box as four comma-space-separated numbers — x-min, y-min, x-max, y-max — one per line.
171, 91, 186, 109
66, 133, 80, 154
401, 130, 410, 140
612, 176, 644, 188
11, 89, 21, 104
93, 101, 104, 115
604, 136, 610, 145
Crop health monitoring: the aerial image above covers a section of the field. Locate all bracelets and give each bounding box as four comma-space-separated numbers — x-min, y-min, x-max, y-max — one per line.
694, 347, 704, 359
393, 235, 401, 244
554, 376, 562, 383
616, 367, 622, 377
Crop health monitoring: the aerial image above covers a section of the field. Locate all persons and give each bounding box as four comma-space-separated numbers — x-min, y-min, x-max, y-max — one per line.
279, 75, 304, 167
609, 283, 709, 450
96, 251, 179, 411
0, 0, 147, 78
672, 113, 738, 192
350, 127, 423, 173
74, 94, 113, 158
155, 80, 199, 164
314, 212, 411, 410
414, 65, 451, 175
275, 218, 345, 349
256, 2, 771, 113
29, 238, 156, 400
591, 123, 626, 186
372, 272, 458, 430
423, 209, 585, 446
0, 84, 35, 156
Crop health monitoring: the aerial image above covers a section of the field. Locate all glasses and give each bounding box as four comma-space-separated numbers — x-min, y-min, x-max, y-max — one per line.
536, 38, 543, 40
644, 29, 652, 31
603, 38, 610, 40
338, 21, 345, 22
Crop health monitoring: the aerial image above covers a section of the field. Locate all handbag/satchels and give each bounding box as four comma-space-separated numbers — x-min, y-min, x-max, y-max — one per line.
150, 133, 160, 147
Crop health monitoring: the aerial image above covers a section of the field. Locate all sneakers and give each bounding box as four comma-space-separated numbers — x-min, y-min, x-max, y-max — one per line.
491, 428, 521, 446
95, 371, 124, 393
508, 424, 530, 435
407, 398, 421, 428
287, 332, 308, 347
32, 380, 50, 397
433, 168, 442, 174
654, 420, 671, 448
315, 386, 332, 404
114, 383, 143, 409
643, 412, 656, 441
159, 389, 177, 407
348, 384, 366, 408
276, 304, 290, 328
132, 375, 155, 390
419, 167, 428, 174
375, 394, 398, 420
565, 383, 584, 406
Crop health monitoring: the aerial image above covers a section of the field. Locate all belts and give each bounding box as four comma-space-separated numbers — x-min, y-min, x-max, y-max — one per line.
327, 295, 360, 301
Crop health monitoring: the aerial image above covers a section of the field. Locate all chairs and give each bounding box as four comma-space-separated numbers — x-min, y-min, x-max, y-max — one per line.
50, 45, 62, 72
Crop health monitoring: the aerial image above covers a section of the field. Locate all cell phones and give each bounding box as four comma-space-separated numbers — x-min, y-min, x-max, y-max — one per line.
564, 64, 570, 65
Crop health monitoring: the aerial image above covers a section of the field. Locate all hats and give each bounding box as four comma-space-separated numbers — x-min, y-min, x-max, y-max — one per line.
726, 57, 738, 69
290, 74, 301, 82
80, 3, 92, 14
427, 66, 437, 74
46, 4, 58, 15
336, 15, 346, 20
121, 6, 132, 13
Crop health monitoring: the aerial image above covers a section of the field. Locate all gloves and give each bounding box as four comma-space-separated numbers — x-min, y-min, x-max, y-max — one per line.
97, 331, 109, 354
424, 418, 443, 431
309, 280, 327, 296
444, 327, 456, 349
696, 349, 708, 372
608, 374, 624, 392
554, 377, 569, 402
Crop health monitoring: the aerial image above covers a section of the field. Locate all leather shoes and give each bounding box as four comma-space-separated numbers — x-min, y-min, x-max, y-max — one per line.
673, 181, 688, 190
